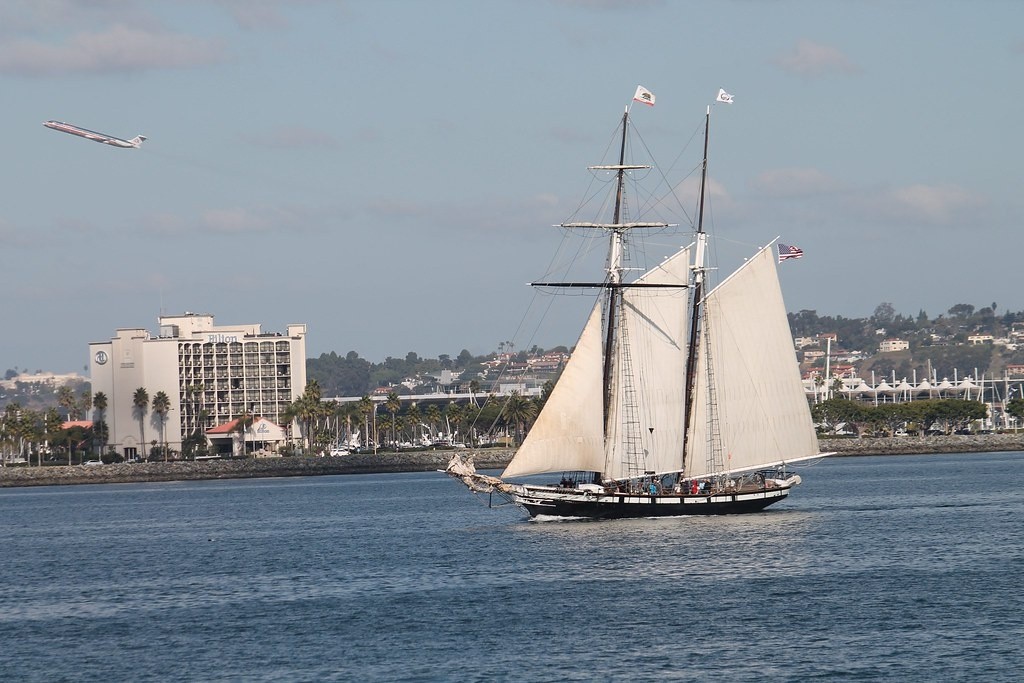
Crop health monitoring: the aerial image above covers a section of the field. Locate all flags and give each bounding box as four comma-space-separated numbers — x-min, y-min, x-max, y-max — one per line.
633, 85, 655, 105
717, 89, 734, 103
778, 244, 803, 264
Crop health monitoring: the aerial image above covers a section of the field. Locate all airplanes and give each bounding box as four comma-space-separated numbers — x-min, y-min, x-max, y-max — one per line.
43, 119, 148, 148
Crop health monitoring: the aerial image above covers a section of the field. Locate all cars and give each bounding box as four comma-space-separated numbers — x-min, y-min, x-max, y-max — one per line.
85, 460, 103, 464
331, 449, 351, 457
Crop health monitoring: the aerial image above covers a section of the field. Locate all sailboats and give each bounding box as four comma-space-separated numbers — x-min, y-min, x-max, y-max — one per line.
439, 86, 820, 517
